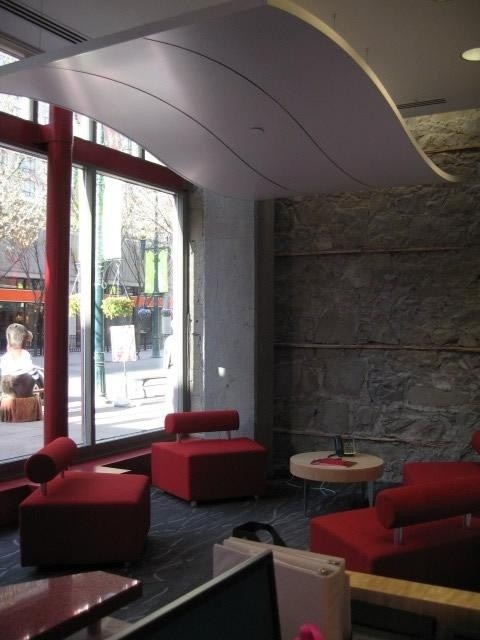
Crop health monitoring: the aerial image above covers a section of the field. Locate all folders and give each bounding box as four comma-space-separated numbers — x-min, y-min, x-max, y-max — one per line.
212, 536, 351, 640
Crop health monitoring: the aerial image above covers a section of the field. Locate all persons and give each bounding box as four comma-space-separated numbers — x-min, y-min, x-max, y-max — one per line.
1, 323, 34, 374
163, 320, 174, 378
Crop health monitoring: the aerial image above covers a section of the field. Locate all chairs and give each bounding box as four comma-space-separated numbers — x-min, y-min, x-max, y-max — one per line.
19, 435, 149, 582
150, 411, 276, 504
308, 477, 480, 576
402, 430, 480, 482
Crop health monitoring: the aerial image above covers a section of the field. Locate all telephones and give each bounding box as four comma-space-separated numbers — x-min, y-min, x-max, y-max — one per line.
334, 434, 356, 457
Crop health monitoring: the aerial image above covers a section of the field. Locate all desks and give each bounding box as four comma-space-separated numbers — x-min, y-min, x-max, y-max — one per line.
0, 571, 142, 640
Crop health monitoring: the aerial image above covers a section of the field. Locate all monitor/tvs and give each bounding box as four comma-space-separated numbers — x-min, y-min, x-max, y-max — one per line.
108, 545, 284, 640
